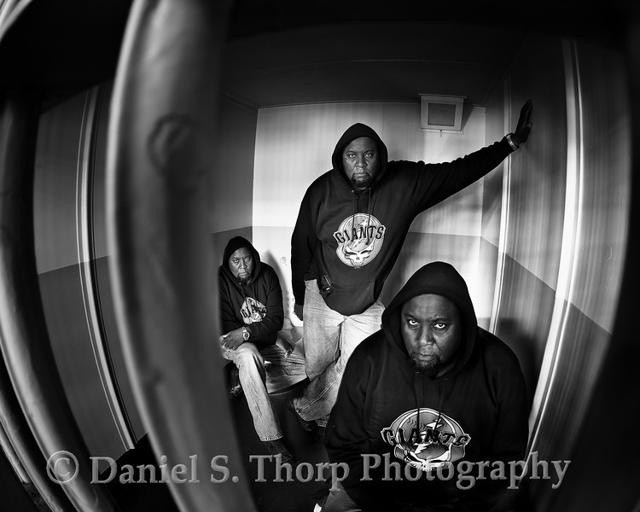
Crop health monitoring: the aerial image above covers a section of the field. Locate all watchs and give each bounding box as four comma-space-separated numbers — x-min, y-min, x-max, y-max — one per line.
241, 326, 251, 342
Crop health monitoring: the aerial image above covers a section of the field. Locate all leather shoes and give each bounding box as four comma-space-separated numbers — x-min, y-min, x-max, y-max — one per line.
264, 439, 297, 472
222, 362, 243, 401
291, 395, 329, 437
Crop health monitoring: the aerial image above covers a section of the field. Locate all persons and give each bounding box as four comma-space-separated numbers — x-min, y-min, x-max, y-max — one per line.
319, 260, 537, 512
214, 234, 303, 470
286, 96, 537, 435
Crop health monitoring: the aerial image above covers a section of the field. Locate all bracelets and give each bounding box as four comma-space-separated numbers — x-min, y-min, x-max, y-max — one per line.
505, 131, 519, 152
